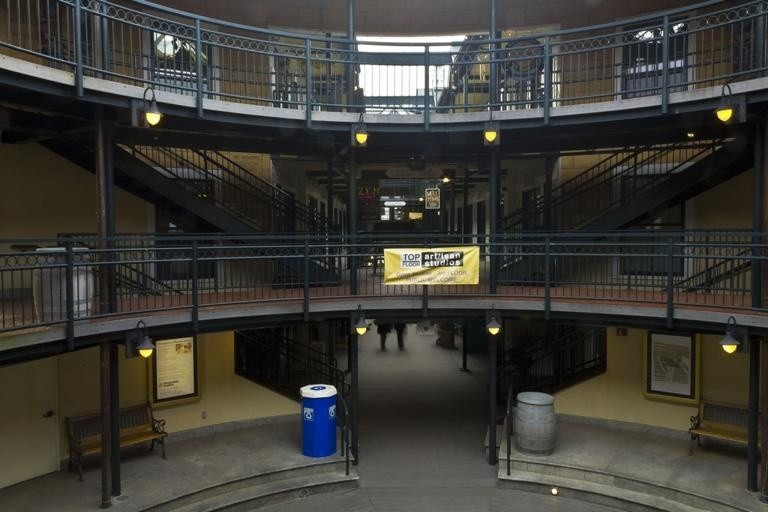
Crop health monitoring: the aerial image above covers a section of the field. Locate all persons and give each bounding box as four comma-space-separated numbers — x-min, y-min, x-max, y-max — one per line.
394, 318, 408, 352
373, 318, 392, 352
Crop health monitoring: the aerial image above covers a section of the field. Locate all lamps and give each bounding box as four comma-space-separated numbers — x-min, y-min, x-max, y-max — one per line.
350, 112, 368, 148
126, 321, 156, 358
130, 87, 162, 128
713, 84, 746, 125
485, 304, 502, 335
718, 316, 741, 354
350, 304, 367, 336
483, 111, 501, 146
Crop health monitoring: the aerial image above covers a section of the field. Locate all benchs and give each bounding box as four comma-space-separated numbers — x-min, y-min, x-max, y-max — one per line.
688, 400, 761, 455
66, 400, 168, 482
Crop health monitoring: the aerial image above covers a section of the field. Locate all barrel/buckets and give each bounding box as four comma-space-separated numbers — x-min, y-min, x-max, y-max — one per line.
31, 246, 95, 321
516, 392, 556, 454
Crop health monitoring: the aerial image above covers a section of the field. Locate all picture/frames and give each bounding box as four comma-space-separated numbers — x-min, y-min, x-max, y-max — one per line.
642, 329, 702, 407
151, 334, 199, 403
